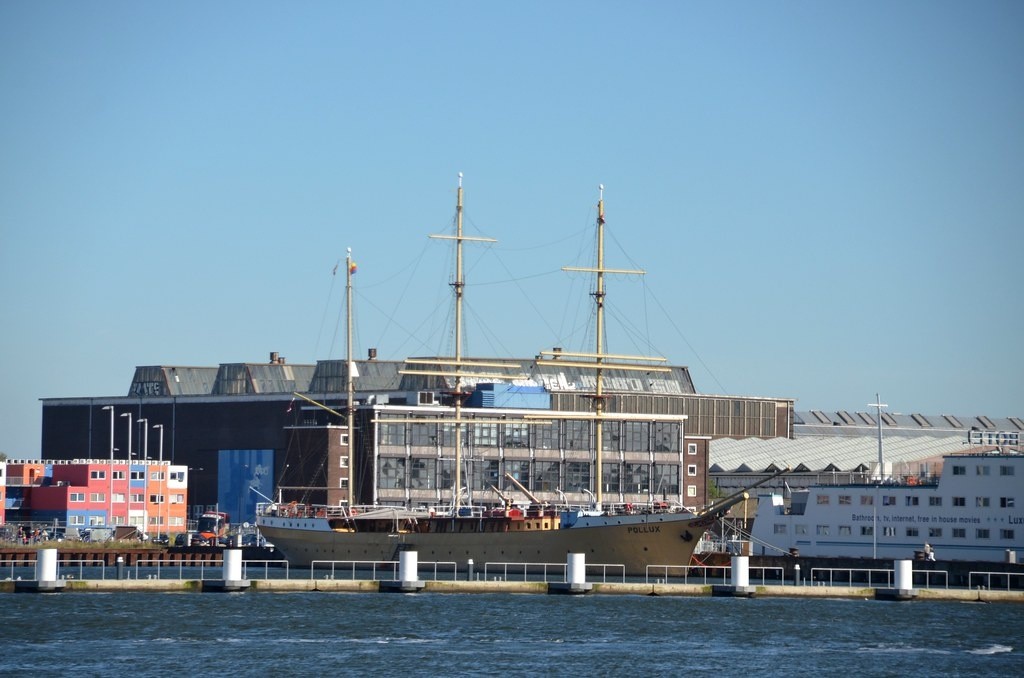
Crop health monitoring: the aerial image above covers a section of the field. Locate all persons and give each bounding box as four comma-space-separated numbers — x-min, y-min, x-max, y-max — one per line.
17, 526, 37, 545
924, 541, 935, 560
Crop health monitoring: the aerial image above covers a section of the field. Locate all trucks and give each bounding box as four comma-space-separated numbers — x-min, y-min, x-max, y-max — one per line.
197, 511, 264, 546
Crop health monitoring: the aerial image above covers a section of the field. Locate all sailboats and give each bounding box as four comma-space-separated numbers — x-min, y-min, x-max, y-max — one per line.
249, 172, 791, 575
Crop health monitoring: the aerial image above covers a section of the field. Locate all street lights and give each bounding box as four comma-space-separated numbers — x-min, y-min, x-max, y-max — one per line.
120, 413, 136, 527
137, 418, 152, 533
153, 425, 163, 539
101, 406, 119, 523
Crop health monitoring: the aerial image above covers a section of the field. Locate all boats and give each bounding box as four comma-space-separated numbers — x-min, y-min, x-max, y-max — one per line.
746, 453, 1023, 563
